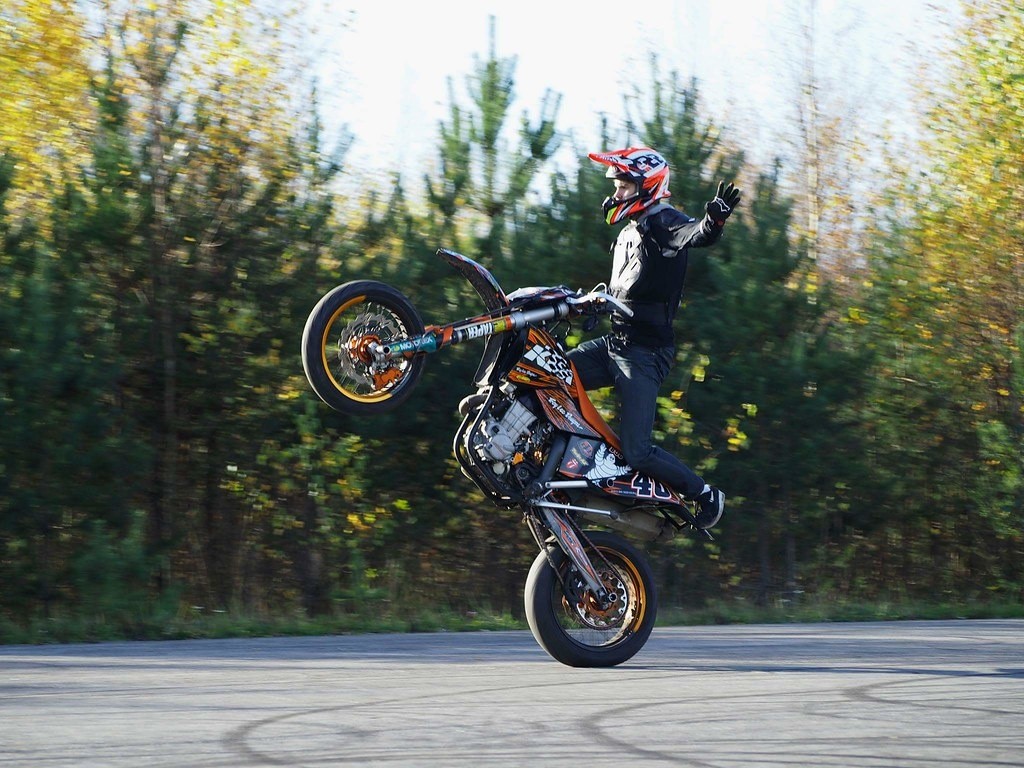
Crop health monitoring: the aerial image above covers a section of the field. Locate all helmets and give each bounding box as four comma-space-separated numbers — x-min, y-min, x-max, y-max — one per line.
588, 147, 672, 225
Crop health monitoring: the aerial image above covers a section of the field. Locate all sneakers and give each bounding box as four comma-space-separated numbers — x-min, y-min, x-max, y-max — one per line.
694, 486, 725, 529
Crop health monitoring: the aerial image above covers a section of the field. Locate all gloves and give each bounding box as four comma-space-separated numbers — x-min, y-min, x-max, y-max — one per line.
707, 181, 740, 227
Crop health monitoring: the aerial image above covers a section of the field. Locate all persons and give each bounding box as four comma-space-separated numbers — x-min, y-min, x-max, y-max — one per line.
461, 148, 741, 529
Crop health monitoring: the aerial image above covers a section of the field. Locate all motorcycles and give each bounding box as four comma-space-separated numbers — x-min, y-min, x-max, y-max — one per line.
300, 248, 714, 668
